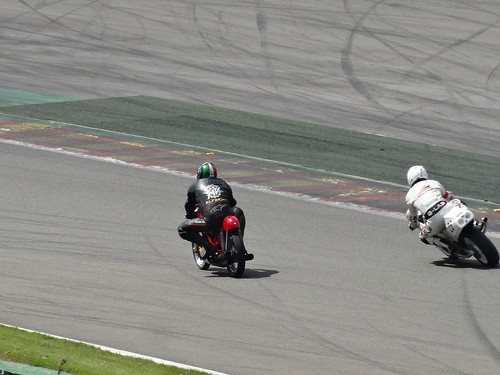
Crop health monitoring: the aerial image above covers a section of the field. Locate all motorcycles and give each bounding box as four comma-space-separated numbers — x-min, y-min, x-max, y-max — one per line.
185, 202, 254, 279
408, 191, 500, 269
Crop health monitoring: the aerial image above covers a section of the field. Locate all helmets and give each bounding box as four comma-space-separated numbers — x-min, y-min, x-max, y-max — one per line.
197, 162, 217, 179
407, 165, 427, 187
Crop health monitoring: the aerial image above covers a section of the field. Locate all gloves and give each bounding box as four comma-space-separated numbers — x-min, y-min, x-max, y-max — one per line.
408, 222, 417, 230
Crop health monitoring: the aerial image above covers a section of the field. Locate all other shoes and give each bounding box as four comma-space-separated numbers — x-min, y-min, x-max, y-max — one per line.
202, 245, 217, 260
433, 238, 449, 249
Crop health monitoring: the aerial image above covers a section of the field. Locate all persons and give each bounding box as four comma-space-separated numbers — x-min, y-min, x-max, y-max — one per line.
403, 164, 478, 253
177, 162, 254, 262
